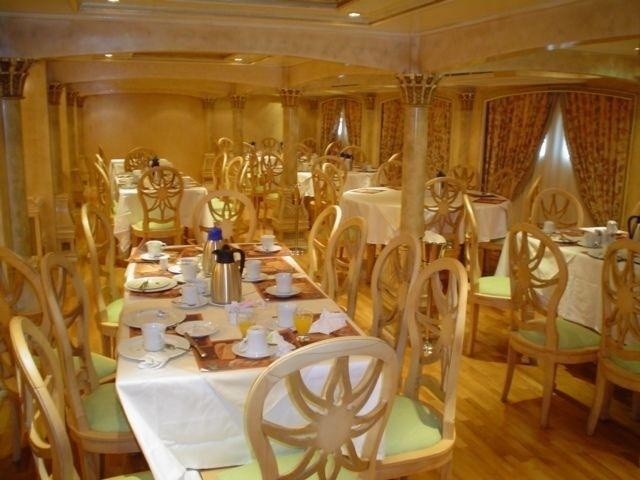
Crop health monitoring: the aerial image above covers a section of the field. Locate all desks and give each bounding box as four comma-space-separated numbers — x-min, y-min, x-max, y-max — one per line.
116, 240, 382, 478
340, 188, 511, 286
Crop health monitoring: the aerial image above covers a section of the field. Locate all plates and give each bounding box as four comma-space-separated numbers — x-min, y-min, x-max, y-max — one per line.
117, 176, 135, 189
116, 245, 302, 361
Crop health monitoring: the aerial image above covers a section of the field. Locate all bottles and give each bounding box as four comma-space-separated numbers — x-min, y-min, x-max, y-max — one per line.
339, 152, 345, 170
436, 171, 446, 197
151, 156, 160, 183
345, 154, 353, 171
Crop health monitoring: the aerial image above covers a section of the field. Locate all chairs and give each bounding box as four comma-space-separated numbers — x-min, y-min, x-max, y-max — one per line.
79, 206, 125, 356
10, 316, 153, 480
3, 248, 119, 395
531, 187, 584, 230
1, 246, 50, 470
326, 216, 367, 321
523, 179, 542, 220
310, 204, 341, 298
0, 135, 525, 242
460, 195, 516, 355
501, 223, 615, 430
372, 256, 468, 478
421, 177, 471, 265
586, 239, 640, 435
368, 233, 418, 391
201, 336, 398, 480
40, 251, 139, 477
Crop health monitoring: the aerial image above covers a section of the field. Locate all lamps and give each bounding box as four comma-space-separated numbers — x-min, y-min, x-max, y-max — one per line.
363, 96, 375, 110
230, 96, 244, 110
459, 92, 475, 109
397, 74, 439, 108
47, 83, 86, 107
203, 98, 216, 108
279, 89, 300, 107
1, 57, 32, 100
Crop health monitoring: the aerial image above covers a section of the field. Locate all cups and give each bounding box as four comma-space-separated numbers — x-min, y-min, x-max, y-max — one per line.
543, 221, 624, 259
140, 235, 293, 354
294, 312, 313, 335
